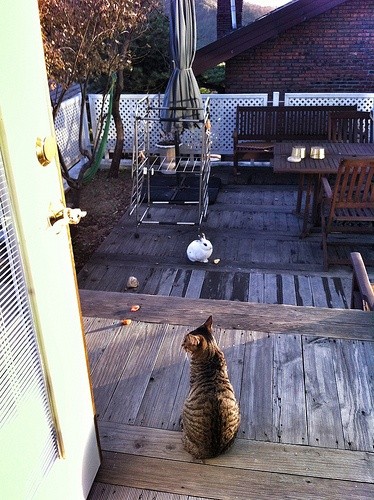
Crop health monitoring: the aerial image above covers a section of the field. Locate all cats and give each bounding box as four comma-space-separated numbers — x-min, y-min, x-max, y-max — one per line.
180, 314, 241, 458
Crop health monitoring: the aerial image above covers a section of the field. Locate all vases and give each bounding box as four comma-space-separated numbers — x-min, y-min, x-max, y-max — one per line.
155, 143, 184, 169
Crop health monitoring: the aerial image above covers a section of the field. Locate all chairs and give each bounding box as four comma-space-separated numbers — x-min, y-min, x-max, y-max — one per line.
329, 111, 374, 143
349, 252, 374, 312
319, 158, 374, 272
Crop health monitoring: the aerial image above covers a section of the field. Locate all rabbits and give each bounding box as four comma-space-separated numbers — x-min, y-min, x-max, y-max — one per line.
186, 233, 213, 263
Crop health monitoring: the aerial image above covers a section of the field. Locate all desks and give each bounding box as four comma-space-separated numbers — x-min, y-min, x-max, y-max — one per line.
274, 143, 374, 239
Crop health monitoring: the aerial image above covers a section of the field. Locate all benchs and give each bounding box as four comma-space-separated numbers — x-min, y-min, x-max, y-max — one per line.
233, 104, 365, 175
